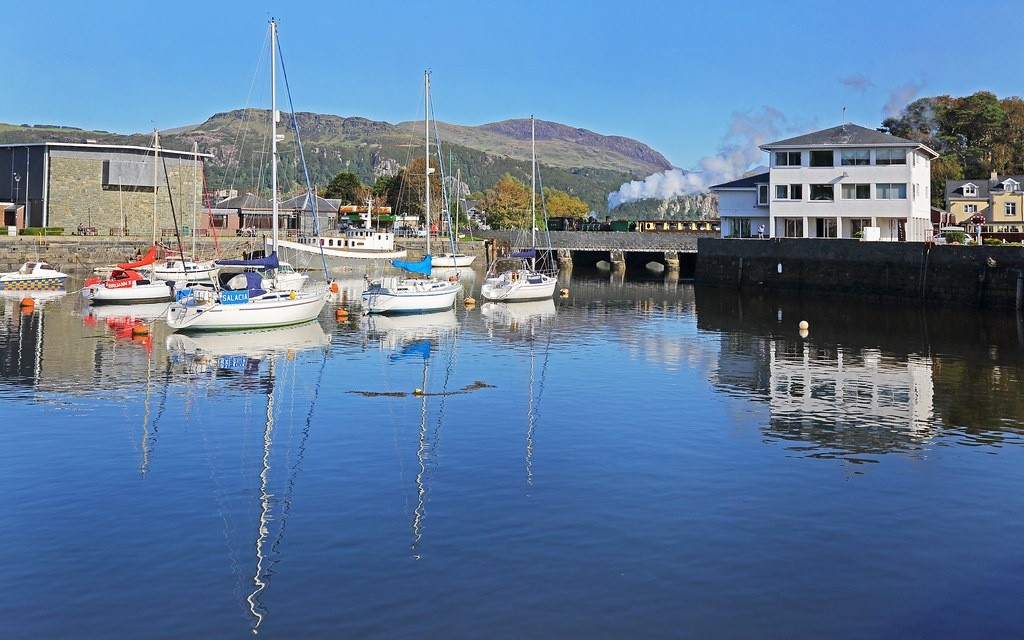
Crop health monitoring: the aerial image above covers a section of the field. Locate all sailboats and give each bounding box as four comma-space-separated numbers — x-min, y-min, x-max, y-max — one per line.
354, 309, 463, 561
164, 321, 332, 636
360, 67, 476, 319
481, 114, 562, 301
479, 299, 566, 487
81, 15, 332, 332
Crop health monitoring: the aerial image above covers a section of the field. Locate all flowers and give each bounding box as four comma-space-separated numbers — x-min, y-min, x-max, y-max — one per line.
970, 211, 986, 227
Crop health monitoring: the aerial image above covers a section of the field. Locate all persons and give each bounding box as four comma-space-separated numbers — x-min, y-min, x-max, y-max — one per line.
403, 223, 419, 238
757, 224, 765, 239
431, 223, 439, 240
77, 223, 86, 236
461, 224, 481, 233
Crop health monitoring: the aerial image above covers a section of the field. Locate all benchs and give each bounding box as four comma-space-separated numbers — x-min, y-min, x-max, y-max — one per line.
161, 227, 177, 236
79, 226, 99, 235
110, 227, 126, 236
191, 229, 209, 237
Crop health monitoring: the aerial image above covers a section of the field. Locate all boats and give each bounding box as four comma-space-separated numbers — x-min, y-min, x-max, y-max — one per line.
0, 238, 69, 289
